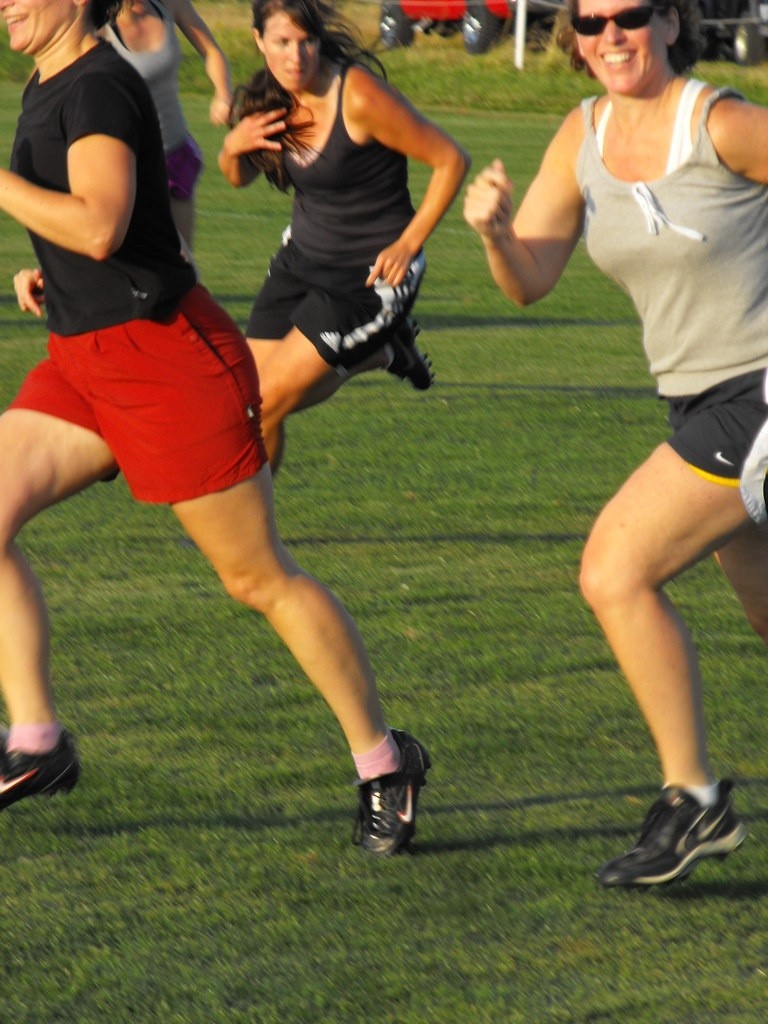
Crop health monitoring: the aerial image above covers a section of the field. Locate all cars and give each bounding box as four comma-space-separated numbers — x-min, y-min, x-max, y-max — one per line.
377, 0, 518, 57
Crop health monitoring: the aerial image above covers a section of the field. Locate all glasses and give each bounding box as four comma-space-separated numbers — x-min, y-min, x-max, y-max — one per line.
570, 7, 666, 36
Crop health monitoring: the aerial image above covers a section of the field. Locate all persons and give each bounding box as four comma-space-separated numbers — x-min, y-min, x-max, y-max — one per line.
463, 1, 768, 889
1, 1, 434, 854
93, 1, 234, 286
216, 0, 470, 481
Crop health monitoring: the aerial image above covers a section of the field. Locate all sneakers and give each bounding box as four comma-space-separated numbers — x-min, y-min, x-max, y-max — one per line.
0, 726, 80, 812
381, 317, 435, 391
600, 780, 748, 890
351, 727, 429, 855
